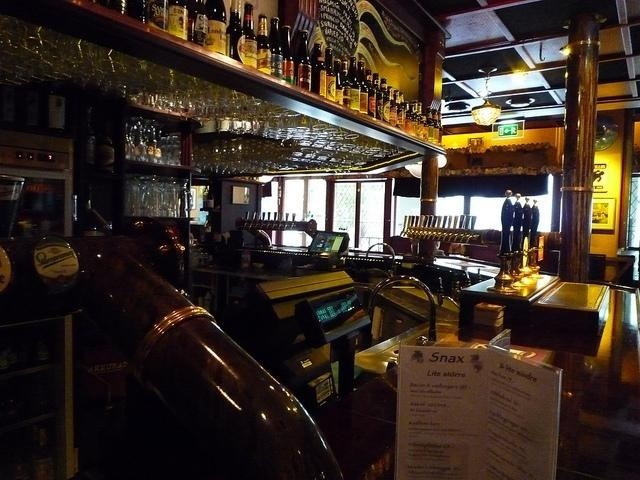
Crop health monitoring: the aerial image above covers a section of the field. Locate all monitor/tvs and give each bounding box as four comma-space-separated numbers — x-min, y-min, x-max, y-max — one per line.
309, 231, 349, 255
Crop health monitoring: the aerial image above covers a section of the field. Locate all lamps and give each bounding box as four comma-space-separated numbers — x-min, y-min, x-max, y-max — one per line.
471, 66, 501, 126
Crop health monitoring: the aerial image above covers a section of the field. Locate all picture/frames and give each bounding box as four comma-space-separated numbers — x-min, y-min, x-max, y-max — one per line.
592, 197, 617, 235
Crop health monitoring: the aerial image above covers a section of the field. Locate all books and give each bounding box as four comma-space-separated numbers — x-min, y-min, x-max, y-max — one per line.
395, 341, 563, 480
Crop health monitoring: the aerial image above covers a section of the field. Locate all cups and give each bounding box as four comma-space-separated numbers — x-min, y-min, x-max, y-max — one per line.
126, 113, 185, 166
124, 173, 189, 217
0, 13, 107, 91
190, 135, 407, 176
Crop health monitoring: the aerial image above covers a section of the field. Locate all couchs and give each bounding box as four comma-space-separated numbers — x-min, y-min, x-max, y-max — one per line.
391, 231, 560, 271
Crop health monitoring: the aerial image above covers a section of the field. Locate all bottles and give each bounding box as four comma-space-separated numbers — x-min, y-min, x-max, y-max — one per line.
403, 215, 477, 242
499, 189, 540, 253
241, 211, 296, 231
109, 0, 444, 147
